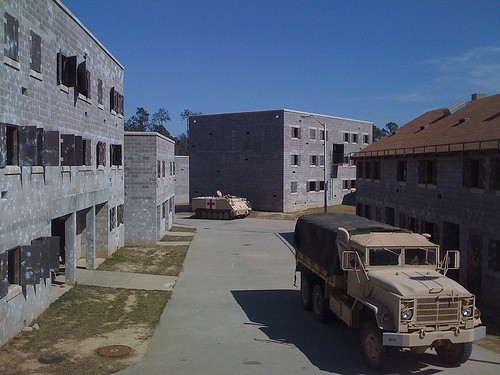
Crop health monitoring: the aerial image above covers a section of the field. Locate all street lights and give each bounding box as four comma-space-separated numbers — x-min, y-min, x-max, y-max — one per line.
301, 114, 327, 213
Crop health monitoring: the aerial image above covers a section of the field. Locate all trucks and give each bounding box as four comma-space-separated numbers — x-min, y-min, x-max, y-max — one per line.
294, 211, 487, 374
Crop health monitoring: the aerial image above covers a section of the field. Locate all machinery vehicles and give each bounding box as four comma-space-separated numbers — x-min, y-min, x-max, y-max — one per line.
192, 191, 253, 220
